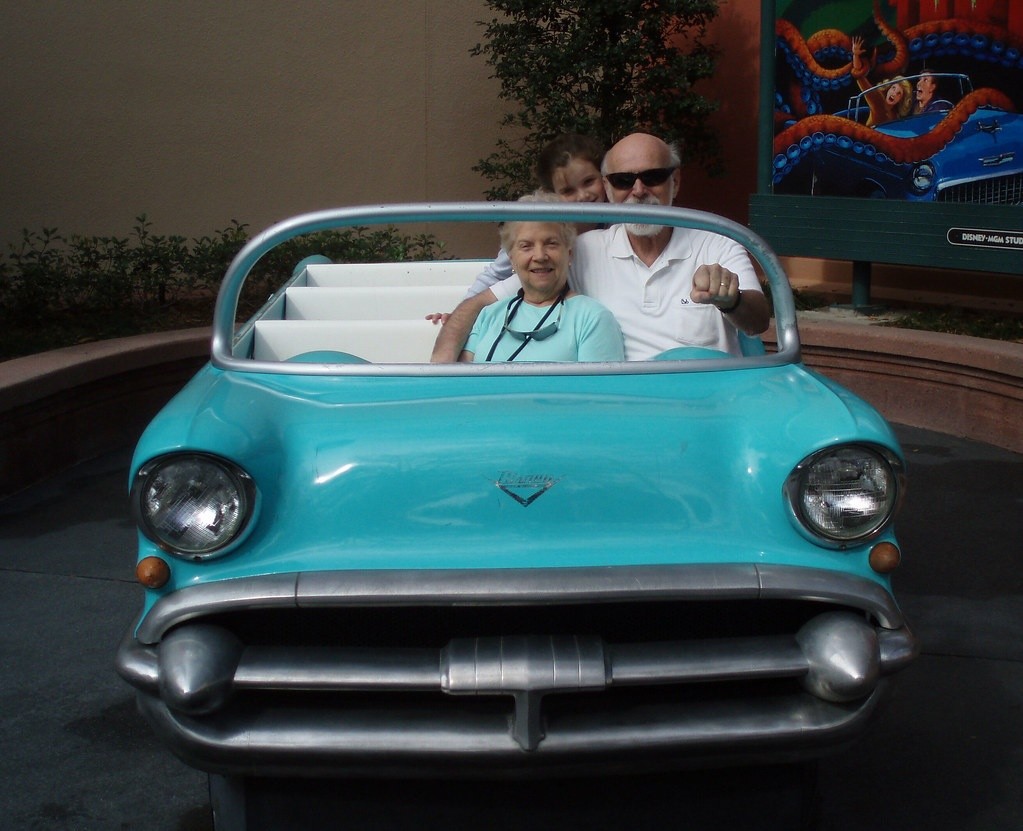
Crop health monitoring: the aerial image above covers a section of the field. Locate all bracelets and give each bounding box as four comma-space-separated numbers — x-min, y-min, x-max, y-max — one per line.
716, 291, 743, 313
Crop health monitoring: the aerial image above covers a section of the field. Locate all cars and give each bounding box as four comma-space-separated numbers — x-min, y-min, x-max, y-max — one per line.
114, 202, 921, 784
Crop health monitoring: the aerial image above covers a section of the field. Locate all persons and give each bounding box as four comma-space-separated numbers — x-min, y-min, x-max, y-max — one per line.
459, 191, 625, 363
425, 138, 610, 324
431, 132, 770, 362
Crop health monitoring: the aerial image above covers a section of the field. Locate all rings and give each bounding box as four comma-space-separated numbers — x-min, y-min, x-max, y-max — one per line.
721, 283, 727, 286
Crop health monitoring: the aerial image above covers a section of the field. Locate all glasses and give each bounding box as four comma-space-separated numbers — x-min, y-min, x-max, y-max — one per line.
504, 295, 563, 339
605, 165, 678, 191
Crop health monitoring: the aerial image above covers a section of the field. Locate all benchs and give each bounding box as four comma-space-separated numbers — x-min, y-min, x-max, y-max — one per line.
285, 285, 485, 318
256, 319, 442, 365
307, 262, 487, 288
